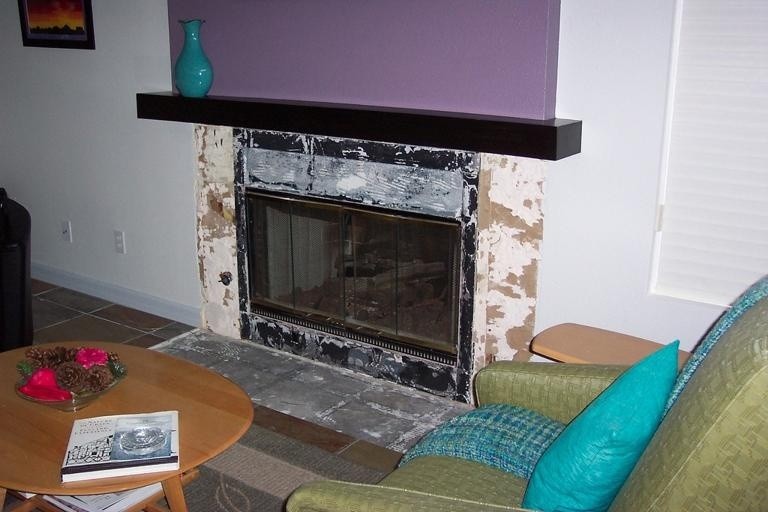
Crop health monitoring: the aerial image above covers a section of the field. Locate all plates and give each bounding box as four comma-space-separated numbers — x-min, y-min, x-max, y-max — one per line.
14, 363, 125, 413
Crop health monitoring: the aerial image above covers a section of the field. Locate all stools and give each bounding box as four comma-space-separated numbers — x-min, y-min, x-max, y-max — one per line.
532, 323, 693, 376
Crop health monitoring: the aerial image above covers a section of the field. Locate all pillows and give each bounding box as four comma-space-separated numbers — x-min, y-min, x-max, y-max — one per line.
521, 340, 681, 511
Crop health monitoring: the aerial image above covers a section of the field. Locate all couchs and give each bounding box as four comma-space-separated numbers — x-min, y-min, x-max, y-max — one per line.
285, 275, 768, 512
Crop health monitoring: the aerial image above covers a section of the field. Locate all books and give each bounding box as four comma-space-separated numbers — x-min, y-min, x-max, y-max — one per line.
44, 480, 164, 512
60, 409, 182, 484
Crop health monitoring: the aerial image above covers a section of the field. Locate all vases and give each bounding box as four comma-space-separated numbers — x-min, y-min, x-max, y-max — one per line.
174, 18, 213, 97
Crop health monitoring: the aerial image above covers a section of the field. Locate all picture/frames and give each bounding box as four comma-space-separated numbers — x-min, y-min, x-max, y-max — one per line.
17, 0, 95, 50
